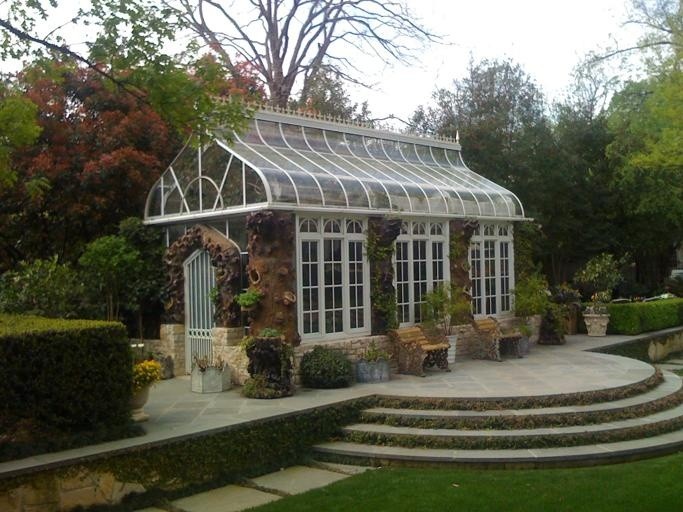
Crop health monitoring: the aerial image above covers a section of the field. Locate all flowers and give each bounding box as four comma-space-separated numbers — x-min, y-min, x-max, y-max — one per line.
132, 358, 162, 394
551, 281, 581, 319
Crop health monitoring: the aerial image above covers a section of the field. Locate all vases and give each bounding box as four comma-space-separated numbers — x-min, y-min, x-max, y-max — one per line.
130, 383, 151, 423
554, 309, 577, 335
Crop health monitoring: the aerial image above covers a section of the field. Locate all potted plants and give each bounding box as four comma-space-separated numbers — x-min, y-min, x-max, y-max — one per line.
156, 355, 174, 380
233, 288, 262, 312
510, 266, 548, 346
356, 337, 393, 382
243, 326, 296, 398
582, 305, 611, 336
190, 350, 234, 393
422, 283, 457, 364
500, 322, 533, 360
